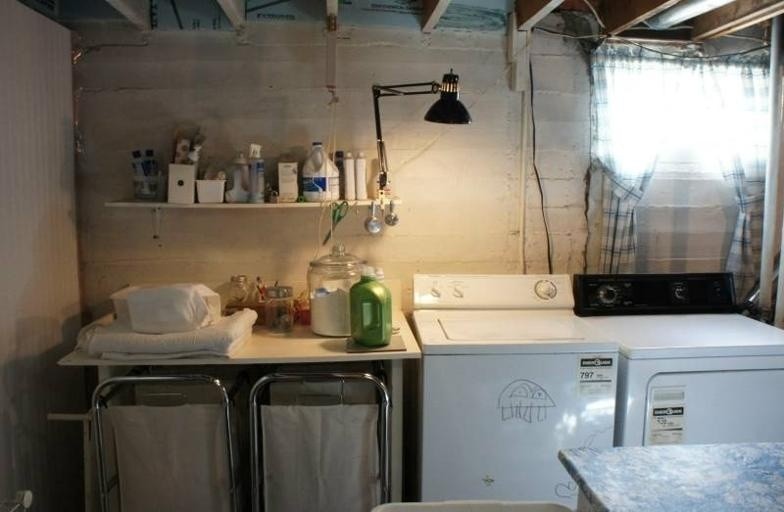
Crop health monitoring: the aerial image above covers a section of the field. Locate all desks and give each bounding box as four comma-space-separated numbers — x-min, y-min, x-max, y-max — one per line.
556, 442, 783, 510
57, 313, 422, 511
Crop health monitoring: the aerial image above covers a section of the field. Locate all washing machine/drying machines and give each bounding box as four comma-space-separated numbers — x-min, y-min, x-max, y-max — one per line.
571, 269, 784, 450
409, 272, 622, 510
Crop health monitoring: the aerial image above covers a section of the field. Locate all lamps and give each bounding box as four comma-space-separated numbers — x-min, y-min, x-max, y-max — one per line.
371, 69, 471, 200
